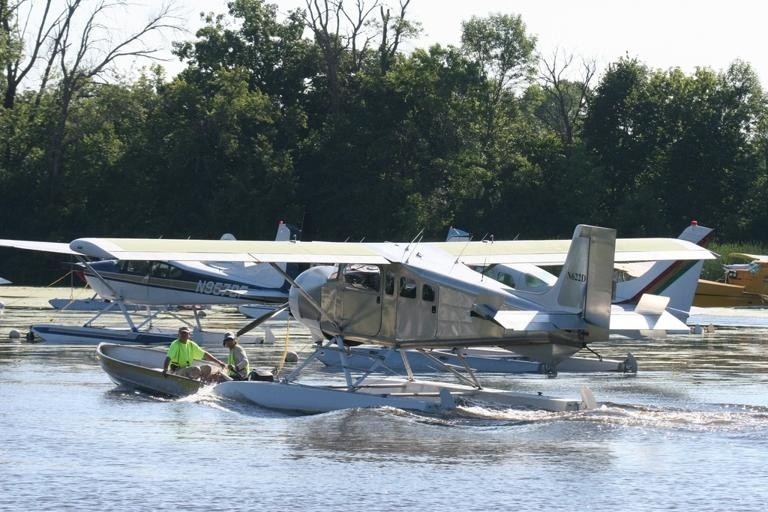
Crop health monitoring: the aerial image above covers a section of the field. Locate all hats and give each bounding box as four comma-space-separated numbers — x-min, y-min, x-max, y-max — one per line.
222, 331, 237, 342
178, 326, 191, 334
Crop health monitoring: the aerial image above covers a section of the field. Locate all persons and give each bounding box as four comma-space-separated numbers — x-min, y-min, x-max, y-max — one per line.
223, 331, 249, 381
162, 327, 225, 378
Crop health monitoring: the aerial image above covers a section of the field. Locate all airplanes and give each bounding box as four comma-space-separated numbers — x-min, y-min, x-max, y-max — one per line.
0, 220, 768, 419
